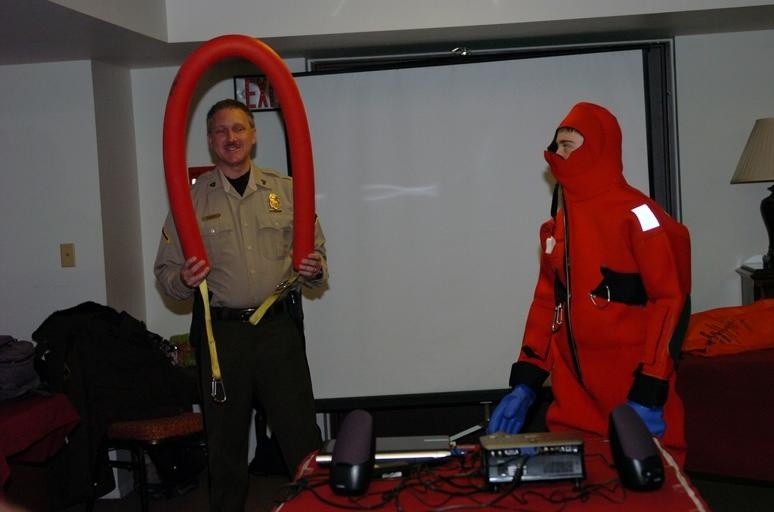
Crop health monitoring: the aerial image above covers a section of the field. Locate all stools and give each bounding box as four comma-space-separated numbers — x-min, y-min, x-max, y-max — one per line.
94, 410, 205, 512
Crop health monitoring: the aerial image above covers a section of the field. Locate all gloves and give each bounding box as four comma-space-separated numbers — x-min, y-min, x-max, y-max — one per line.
487, 384, 536, 434
626, 400, 666, 438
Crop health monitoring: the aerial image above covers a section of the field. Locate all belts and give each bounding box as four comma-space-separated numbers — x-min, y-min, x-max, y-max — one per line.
210, 295, 297, 323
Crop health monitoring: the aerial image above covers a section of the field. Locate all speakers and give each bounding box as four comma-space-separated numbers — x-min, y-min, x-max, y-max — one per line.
329, 408, 376, 496
607, 404, 665, 492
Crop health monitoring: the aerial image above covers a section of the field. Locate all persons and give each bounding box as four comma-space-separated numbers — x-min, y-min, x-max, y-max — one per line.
154, 99, 329, 512
487, 102, 691, 468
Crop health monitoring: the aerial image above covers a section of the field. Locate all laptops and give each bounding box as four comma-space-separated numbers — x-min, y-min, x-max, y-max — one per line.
315, 435, 456, 466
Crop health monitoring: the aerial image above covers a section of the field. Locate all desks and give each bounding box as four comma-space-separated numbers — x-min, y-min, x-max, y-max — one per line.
0, 392, 80, 494
265, 435, 713, 512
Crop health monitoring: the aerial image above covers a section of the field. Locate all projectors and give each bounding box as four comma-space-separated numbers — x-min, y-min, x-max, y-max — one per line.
478, 432, 586, 485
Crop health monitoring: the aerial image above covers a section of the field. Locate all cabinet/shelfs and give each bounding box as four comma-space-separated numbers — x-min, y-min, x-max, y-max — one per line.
735, 250, 773, 309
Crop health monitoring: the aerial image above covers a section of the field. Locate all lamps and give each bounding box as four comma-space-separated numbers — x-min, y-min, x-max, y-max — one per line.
728, 116, 773, 262
